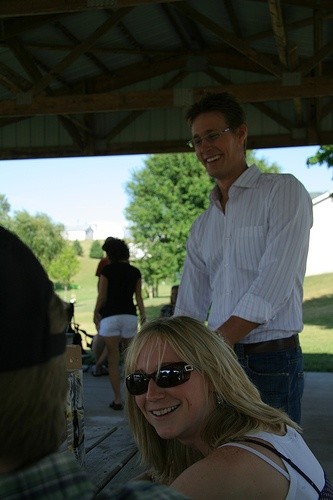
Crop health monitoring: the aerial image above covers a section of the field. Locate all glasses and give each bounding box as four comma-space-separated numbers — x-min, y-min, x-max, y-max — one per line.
124, 361, 194, 395
188, 127, 231, 148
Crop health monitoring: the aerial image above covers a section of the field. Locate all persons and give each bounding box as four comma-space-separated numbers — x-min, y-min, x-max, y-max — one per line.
0, 225, 178, 500
174, 95, 313, 429
125, 316, 333, 500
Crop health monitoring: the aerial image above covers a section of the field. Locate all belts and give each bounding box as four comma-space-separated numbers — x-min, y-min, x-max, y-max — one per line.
243, 334, 299, 354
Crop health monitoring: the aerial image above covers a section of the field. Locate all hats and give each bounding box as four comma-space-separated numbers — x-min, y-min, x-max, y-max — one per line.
0, 225, 68, 374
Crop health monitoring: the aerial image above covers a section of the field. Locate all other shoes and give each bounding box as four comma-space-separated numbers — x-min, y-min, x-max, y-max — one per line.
91, 367, 103, 377
109, 400, 123, 410
